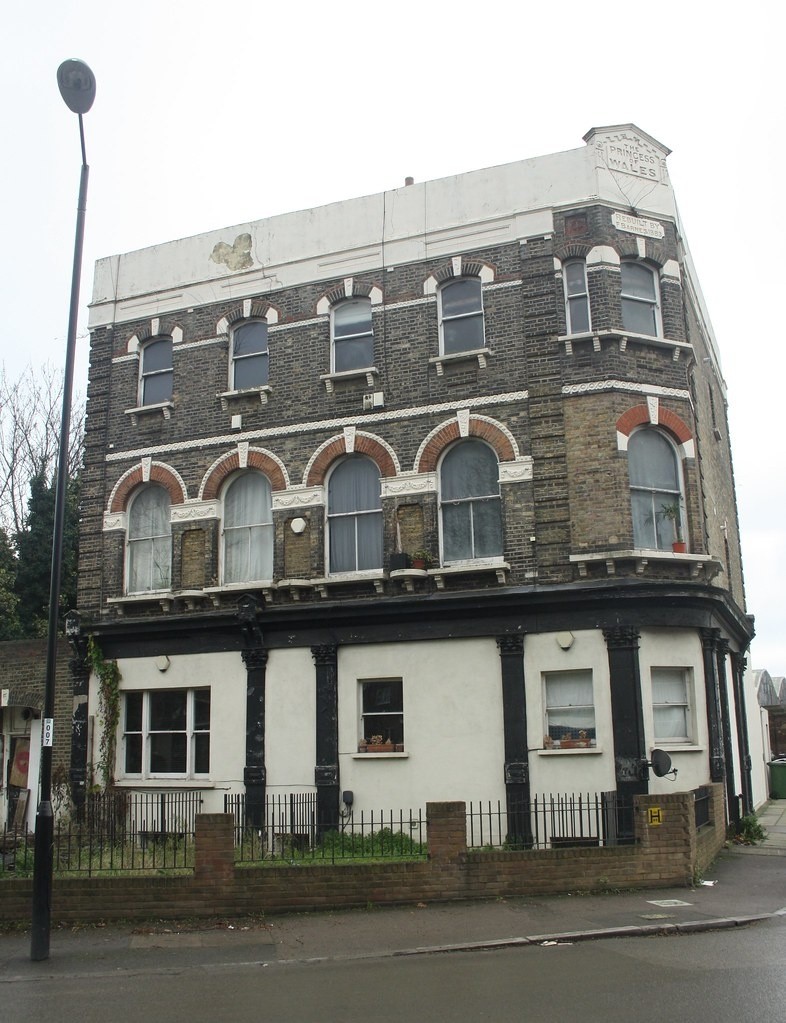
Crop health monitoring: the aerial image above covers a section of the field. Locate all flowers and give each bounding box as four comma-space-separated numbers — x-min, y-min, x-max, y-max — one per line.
370, 735, 392, 744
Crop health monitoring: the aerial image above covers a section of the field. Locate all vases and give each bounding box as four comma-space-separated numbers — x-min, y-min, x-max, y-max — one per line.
367, 744, 396, 752
396, 743, 404, 751
390, 553, 410, 573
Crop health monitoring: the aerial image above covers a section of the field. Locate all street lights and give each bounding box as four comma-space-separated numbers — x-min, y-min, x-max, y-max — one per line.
27, 58, 97, 963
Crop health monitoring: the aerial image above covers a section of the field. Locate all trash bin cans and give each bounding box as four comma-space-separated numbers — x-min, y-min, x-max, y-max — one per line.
766, 758, 786, 800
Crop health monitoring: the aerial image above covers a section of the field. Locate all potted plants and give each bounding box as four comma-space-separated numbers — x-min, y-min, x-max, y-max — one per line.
560, 733, 573, 749
658, 500, 687, 553
577, 730, 592, 747
358, 739, 368, 752
411, 548, 432, 569
544, 735, 554, 749
